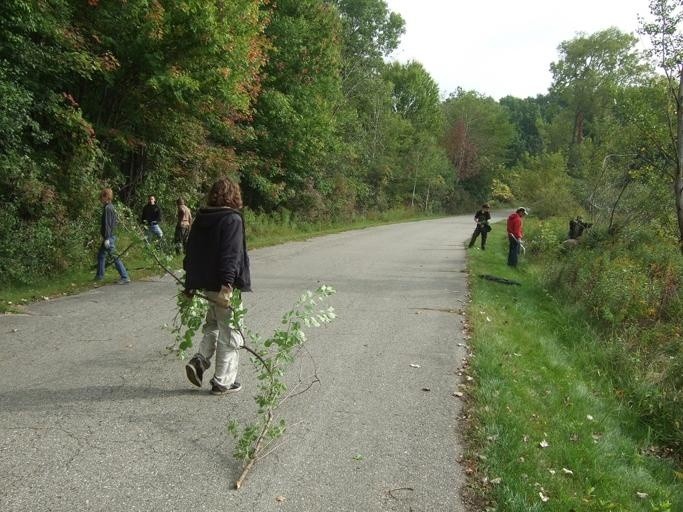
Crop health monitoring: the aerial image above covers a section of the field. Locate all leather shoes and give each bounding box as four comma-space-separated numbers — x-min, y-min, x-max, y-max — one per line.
217, 285, 233, 308
105, 240, 110, 248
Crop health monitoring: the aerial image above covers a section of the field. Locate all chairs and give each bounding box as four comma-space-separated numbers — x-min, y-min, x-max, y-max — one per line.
118, 278, 129, 284
186, 356, 204, 387
212, 382, 242, 394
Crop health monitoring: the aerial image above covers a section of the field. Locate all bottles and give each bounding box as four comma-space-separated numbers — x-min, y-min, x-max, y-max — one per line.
518, 207, 528, 215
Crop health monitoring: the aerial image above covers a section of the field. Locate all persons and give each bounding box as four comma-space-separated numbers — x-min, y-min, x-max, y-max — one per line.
505, 206, 528, 266
176, 177, 249, 395
172, 198, 193, 256
89, 188, 130, 286
140, 195, 163, 242
466, 202, 490, 251
